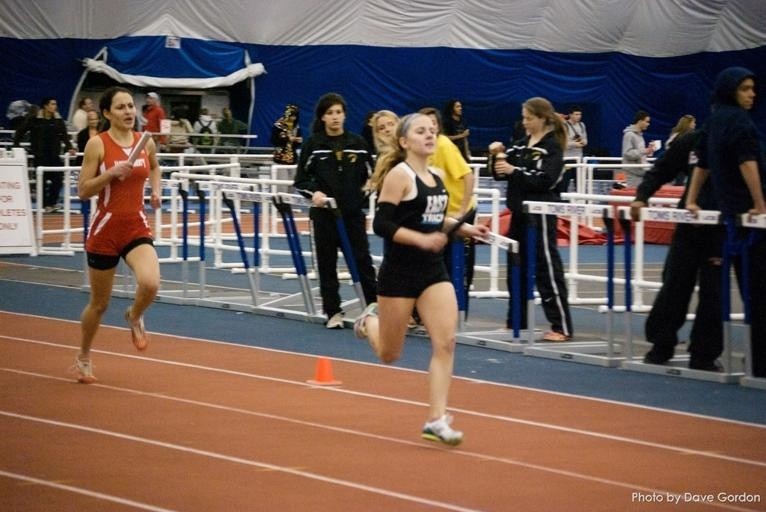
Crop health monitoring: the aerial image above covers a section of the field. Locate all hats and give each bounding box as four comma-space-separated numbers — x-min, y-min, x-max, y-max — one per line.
145, 91, 159, 99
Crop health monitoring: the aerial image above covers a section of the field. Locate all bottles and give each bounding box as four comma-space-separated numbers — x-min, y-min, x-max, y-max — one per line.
495, 149, 509, 179
648, 143, 652, 157
568, 179, 576, 192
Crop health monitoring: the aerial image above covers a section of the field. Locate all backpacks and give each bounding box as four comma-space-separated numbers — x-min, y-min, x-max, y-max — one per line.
197, 119, 213, 150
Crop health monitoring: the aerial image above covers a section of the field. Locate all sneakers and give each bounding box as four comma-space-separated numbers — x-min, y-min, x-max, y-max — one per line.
544, 331, 571, 341
45, 205, 64, 215
326, 310, 346, 329
421, 412, 465, 445
406, 314, 418, 329
689, 357, 724, 371
124, 304, 149, 351
354, 302, 379, 339
68, 353, 98, 384
646, 349, 667, 363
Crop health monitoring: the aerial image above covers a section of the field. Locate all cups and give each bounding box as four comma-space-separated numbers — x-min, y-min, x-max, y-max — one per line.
655, 141, 661, 150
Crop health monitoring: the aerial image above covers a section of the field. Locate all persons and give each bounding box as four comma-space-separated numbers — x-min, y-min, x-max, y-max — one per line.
293, 93, 378, 331
557, 106, 589, 198
70, 85, 163, 383
486, 96, 575, 342
359, 95, 476, 330
270, 103, 303, 165
5, 92, 248, 214
622, 109, 659, 192
686, 67, 765, 377
346, 113, 465, 447
664, 114, 696, 185
628, 119, 729, 375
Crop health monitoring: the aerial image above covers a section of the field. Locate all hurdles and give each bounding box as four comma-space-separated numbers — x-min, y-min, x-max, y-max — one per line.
34, 178, 525, 354
519, 201, 766, 394
